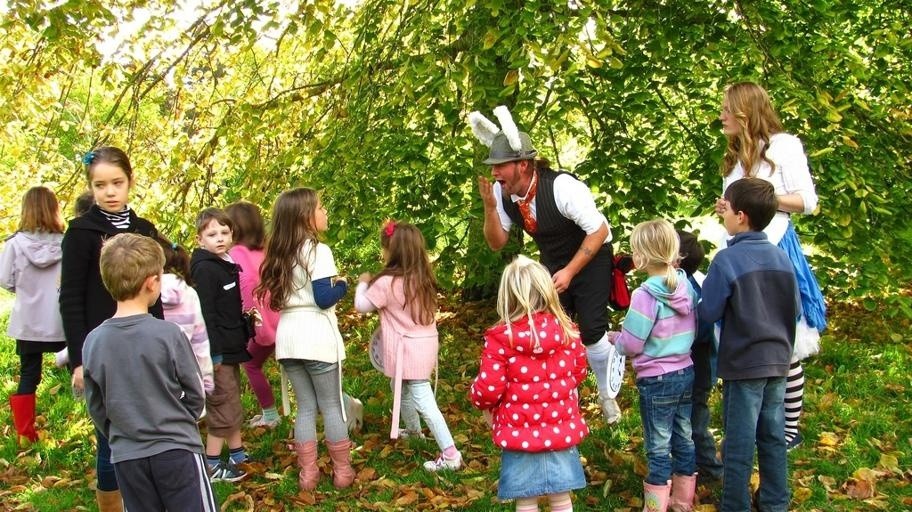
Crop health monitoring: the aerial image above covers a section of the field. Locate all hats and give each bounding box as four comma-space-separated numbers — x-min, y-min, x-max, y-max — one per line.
467, 105, 539, 166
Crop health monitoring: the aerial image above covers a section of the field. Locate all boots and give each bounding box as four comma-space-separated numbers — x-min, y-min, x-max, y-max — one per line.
640, 480, 673, 512
324, 437, 356, 490
666, 473, 698, 512
9, 392, 40, 447
293, 440, 322, 490
94, 481, 124, 512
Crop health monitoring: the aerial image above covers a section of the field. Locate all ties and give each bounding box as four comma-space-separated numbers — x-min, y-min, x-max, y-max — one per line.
518, 176, 538, 234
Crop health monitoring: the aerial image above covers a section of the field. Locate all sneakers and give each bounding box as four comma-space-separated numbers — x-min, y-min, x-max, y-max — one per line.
208, 460, 248, 484
597, 395, 621, 421
252, 417, 283, 430
424, 451, 463, 470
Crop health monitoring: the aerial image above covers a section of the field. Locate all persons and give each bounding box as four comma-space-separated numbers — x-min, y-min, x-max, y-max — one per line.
715, 82, 827, 453
82, 232, 218, 512
223, 202, 282, 429
53, 236, 215, 419
251, 188, 356, 491
355, 221, 462, 472
189, 208, 256, 483
478, 129, 621, 424
607, 219, 698, 512
672, 229, 724, 481
70, 192, 96, 219
470, 255, 588, 512
58, 146, 166, 512
0, 186, 67, 449
698, 178, 797, 512
